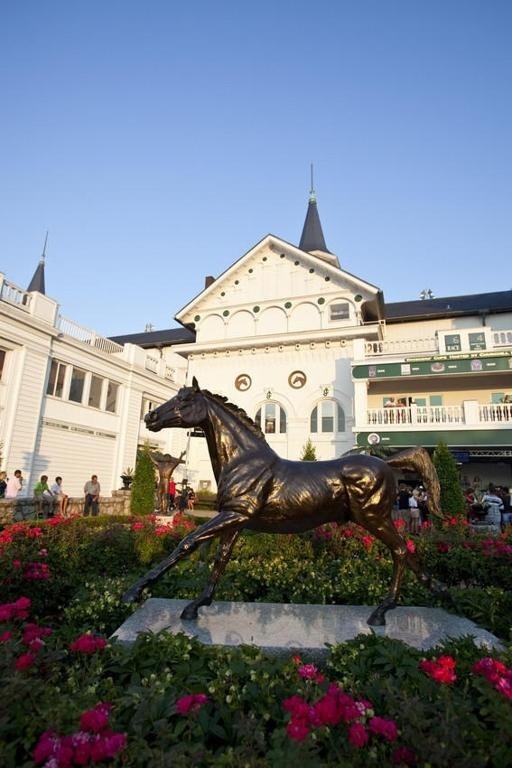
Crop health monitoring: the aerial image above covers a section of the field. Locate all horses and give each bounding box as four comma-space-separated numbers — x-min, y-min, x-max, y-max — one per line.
121, 375, 446, 626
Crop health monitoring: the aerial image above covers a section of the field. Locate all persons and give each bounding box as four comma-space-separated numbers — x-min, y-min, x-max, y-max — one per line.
392, 476, 511, 535
396, 399, 406, 424
502, 395, 510, 421
1, 469, 198, 517
383, 396, 397, 424
